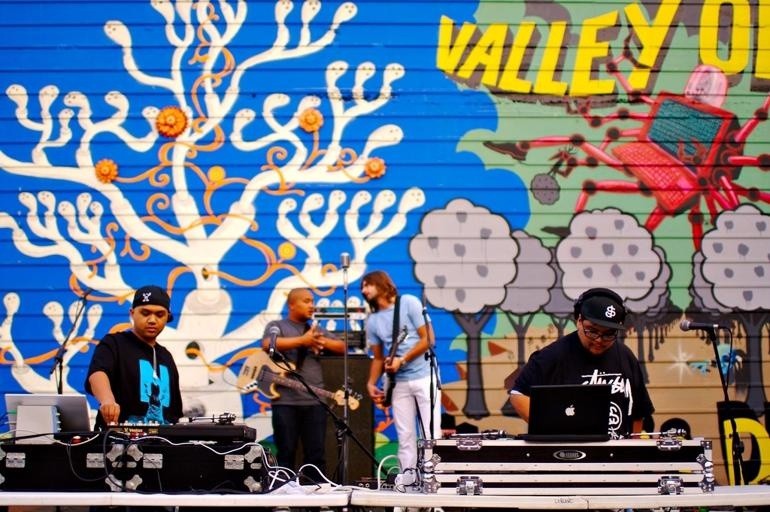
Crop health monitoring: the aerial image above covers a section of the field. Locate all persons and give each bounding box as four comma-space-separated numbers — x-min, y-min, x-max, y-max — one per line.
507, 287, 654, 439
84, 286, 183, 432
359, 271, 443, 475
262, 287, 348, 486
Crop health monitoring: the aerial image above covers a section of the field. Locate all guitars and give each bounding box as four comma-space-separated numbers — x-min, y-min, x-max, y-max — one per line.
236, 350, 361, 411
378, 325, 409, 404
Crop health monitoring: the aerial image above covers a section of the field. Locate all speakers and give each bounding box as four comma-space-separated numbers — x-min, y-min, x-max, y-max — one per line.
321, 354, 375, 486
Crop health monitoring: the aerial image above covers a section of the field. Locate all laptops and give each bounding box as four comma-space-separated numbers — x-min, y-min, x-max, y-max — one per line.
517, 384, 611, 442
4, 394, 92, 437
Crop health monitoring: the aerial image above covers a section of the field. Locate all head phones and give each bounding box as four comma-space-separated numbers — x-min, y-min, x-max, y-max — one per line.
573, 287, 627, 325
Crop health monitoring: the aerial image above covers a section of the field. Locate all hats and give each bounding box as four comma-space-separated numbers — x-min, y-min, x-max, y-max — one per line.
581, 297, 625, 330
132, 286, 174, 323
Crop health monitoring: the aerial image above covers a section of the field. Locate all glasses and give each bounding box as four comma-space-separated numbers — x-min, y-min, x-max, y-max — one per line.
581, 320, 618, 342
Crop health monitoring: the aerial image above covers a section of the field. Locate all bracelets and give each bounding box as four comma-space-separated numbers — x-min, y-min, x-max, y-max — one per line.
399, 356, 406, 368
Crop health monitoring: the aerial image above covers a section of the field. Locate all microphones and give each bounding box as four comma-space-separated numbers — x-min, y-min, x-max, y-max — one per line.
269, 326, 280, 353
340, 252, 350, 270
420, 288, 428, 316
79, 287, 93, 298
679, 319, 729, 332
